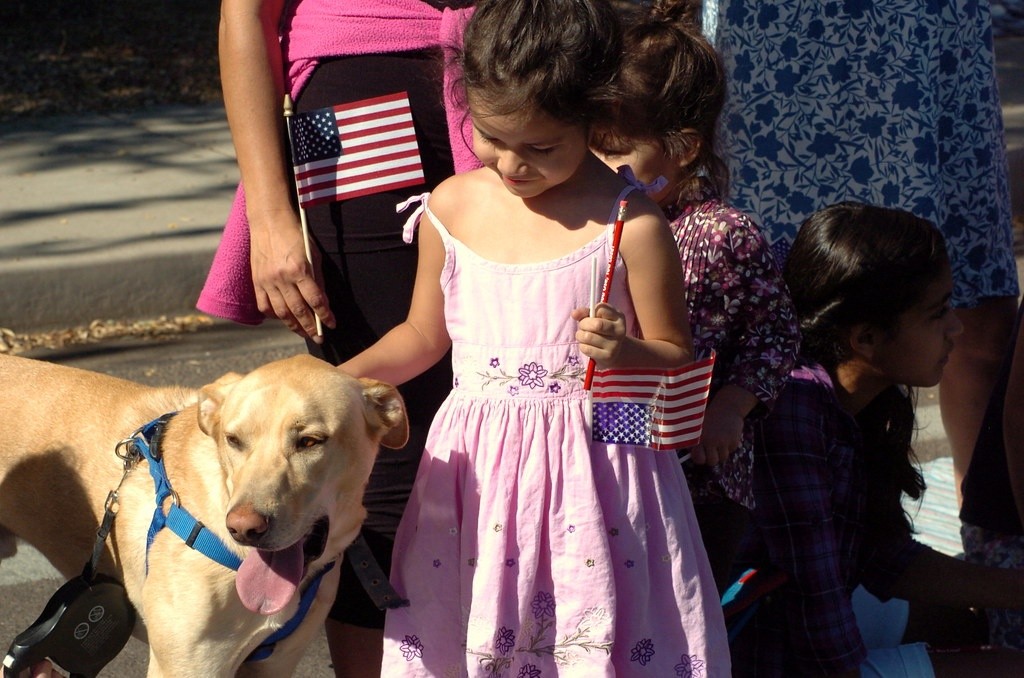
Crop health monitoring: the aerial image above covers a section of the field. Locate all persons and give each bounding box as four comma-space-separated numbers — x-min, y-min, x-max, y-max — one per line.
191, 0, 1024, 677
341, 2, 735, 678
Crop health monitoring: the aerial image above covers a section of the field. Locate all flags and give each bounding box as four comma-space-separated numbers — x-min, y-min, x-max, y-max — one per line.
592, 348, 717, 449
289, 90, 428, 205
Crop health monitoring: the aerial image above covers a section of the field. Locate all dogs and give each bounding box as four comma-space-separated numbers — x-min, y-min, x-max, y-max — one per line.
0, 354, 409, 678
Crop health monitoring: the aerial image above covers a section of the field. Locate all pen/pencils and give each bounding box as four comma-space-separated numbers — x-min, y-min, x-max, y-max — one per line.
579, 198, 630, 392
